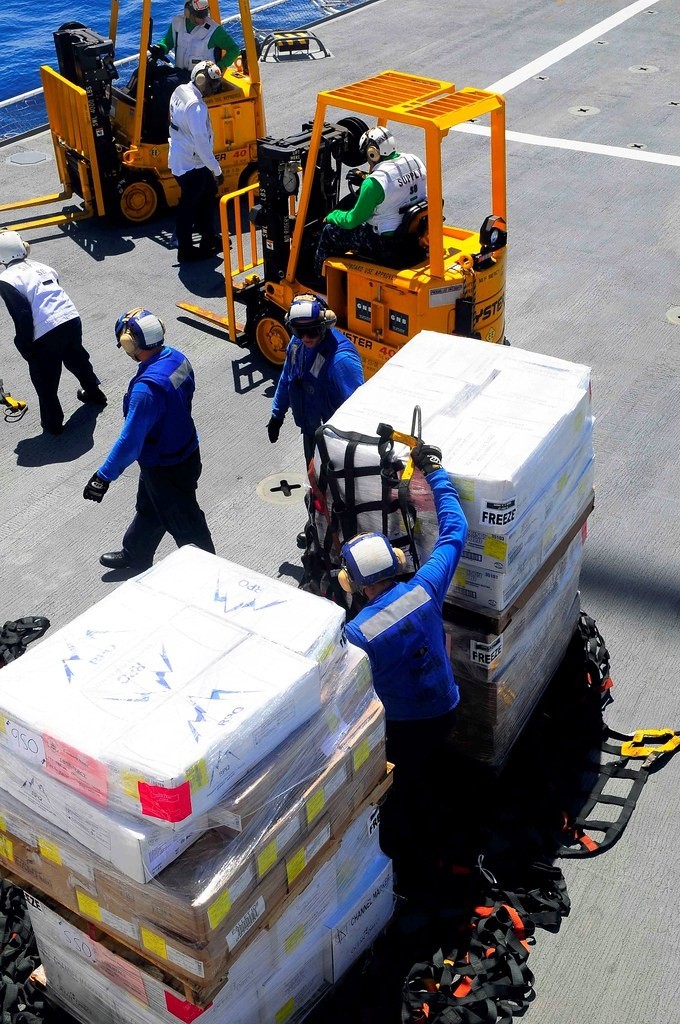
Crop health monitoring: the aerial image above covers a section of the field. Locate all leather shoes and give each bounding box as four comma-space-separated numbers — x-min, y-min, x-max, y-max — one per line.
99, 549, 147, 569
201, 234, 232, 250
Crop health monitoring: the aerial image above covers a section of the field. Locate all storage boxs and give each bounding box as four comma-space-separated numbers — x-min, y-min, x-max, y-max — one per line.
0, 329, 598, 1024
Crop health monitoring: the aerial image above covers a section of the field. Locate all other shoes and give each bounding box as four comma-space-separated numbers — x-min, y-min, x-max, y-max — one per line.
178, 247, 210, 262
77, 389, 107, 406
42, 422, 65, 436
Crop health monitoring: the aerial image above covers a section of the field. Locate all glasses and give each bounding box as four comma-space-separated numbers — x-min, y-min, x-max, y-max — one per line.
291, 324, 324, 339
195, 10, 209, 18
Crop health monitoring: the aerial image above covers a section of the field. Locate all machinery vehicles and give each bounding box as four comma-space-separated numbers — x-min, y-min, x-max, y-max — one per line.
0, 0, 268, 234
176, 70, 509, 385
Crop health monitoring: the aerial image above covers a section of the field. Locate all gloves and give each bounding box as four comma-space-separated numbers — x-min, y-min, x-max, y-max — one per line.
216, 175, 224, 186
148, 44, 164, 55
411, 444, 442, 476
265, 418, 282, 443
346, 168, 363, 185
83, 473, 108, 502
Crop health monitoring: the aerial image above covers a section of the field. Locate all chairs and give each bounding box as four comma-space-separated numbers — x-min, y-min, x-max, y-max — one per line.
360, 199, 445, 269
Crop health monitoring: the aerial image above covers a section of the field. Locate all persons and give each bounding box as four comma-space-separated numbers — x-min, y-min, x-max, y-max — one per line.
0, 229, 106, 434
266, 293, 364, 478
313, 126, 425, 284
154, 0, 240, 98
168, 61, 232, 267
82, 308, 216, 569
340, 445, 468, 800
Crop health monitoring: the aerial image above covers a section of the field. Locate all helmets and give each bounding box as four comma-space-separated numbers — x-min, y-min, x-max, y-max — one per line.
191, 61, 223, 90
0, 231, 26, 264
288, 293, 328, 323
184, 0, 210, 17
341, 532, 398, 585
115, 308, 164, 350
359, 126, 396, 156
